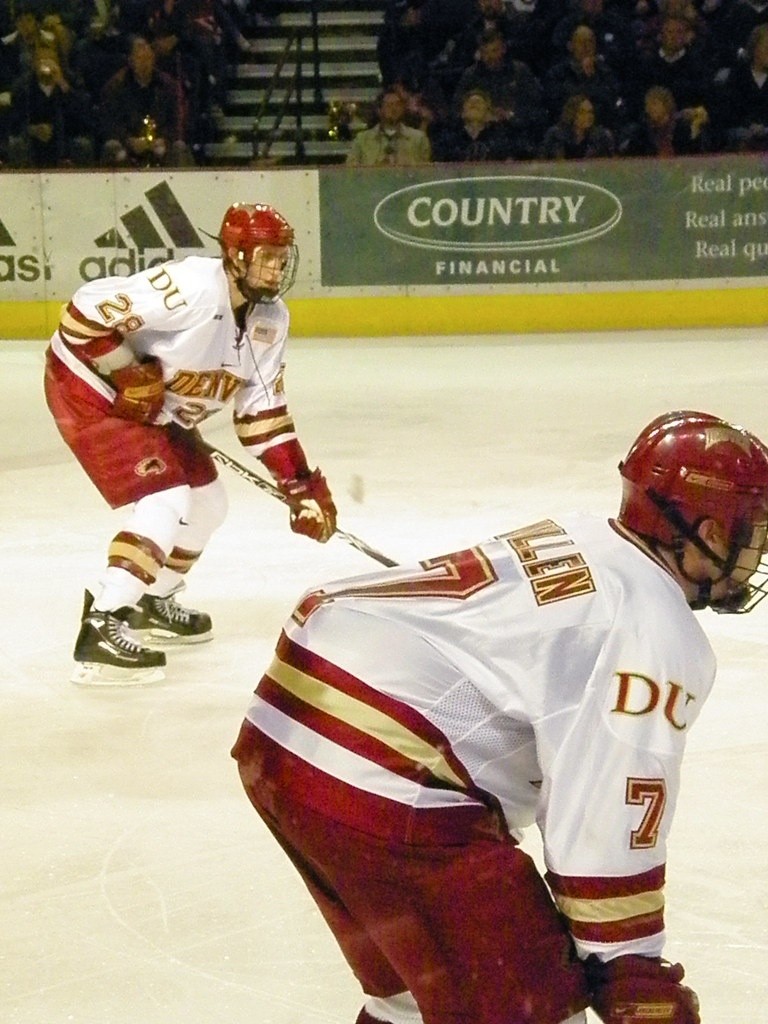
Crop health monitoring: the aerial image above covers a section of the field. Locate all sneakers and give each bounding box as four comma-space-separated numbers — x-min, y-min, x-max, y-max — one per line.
120, 580, 215, 645
70, 589, 167, 686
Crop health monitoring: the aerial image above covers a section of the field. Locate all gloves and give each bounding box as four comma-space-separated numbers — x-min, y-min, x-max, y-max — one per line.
277, 468, 336, 543
590, 954, 700, 1024
111, 355, 163, 424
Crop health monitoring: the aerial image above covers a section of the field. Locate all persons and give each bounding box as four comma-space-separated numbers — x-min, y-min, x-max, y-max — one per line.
0, 0, 257, 168
346, 0, 768, 166
231, 410, 768, 1023
44, 204, 336, 687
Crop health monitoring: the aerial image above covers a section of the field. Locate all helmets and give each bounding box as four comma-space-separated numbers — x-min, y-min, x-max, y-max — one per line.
218, 201, 297, 304
616, 412, 768, 614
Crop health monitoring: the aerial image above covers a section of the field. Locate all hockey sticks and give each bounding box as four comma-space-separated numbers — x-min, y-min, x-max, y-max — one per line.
171, 423, 399, 568
